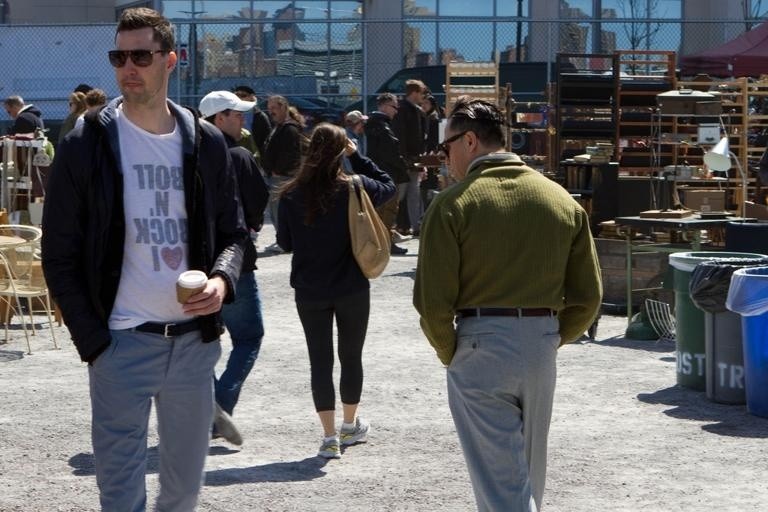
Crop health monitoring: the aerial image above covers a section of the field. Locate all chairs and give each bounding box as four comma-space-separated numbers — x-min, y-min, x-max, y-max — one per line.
0, 240, 60, 355
0, 223, 43, 344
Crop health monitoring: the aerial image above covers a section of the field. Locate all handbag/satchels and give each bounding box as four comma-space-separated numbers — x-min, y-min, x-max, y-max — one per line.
349, 191, 392, 279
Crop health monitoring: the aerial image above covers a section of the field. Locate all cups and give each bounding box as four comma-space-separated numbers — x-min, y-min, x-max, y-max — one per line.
176, 270, 208, 308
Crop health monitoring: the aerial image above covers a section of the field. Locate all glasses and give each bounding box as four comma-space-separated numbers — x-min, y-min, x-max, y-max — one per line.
437, 131, 479, 157
107, 48, 171, 68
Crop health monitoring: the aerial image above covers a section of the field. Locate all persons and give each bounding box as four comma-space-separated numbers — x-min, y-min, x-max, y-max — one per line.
345, 79, 449, 256
186, 88, 271, 446
42, 2, 247, 512
410, 94, 604, 512
236, 89, 307, 254
5, 82, 107, 208
276, 122, 401, 459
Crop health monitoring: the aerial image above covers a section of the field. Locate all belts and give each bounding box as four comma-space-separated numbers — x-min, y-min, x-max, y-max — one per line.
128, 318, 200, 339
455, 307, 556, 324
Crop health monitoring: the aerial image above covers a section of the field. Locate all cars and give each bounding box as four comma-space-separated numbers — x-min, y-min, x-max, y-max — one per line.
253, 95, 350, 128
577, 67, 681, 77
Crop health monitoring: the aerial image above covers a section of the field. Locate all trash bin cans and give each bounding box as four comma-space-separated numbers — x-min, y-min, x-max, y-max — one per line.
724, 267, 768, 418
668, 251, 768, 391
689, 260, 768, 404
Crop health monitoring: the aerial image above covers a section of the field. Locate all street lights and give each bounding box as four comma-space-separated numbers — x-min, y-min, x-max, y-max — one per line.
315, 70, 339, 102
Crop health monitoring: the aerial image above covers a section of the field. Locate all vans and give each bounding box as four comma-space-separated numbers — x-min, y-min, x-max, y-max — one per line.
345, 60, 577, 132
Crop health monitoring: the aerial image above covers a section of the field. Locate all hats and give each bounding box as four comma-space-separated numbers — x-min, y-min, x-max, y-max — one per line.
197, 90, 257, 120
343, 110, 369, 125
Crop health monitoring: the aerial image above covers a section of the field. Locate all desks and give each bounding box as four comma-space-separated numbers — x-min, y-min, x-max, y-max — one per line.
0, 235, 27, 286
612, 215, 758, 325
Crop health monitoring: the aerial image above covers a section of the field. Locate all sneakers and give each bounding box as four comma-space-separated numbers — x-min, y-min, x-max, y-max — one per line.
390, 230, 413, 243
215, 401, 243, 446
392, 243, 407, 254
318, 439, 341, 459
340, 416, 370, 446
265, 242, 286, 254
